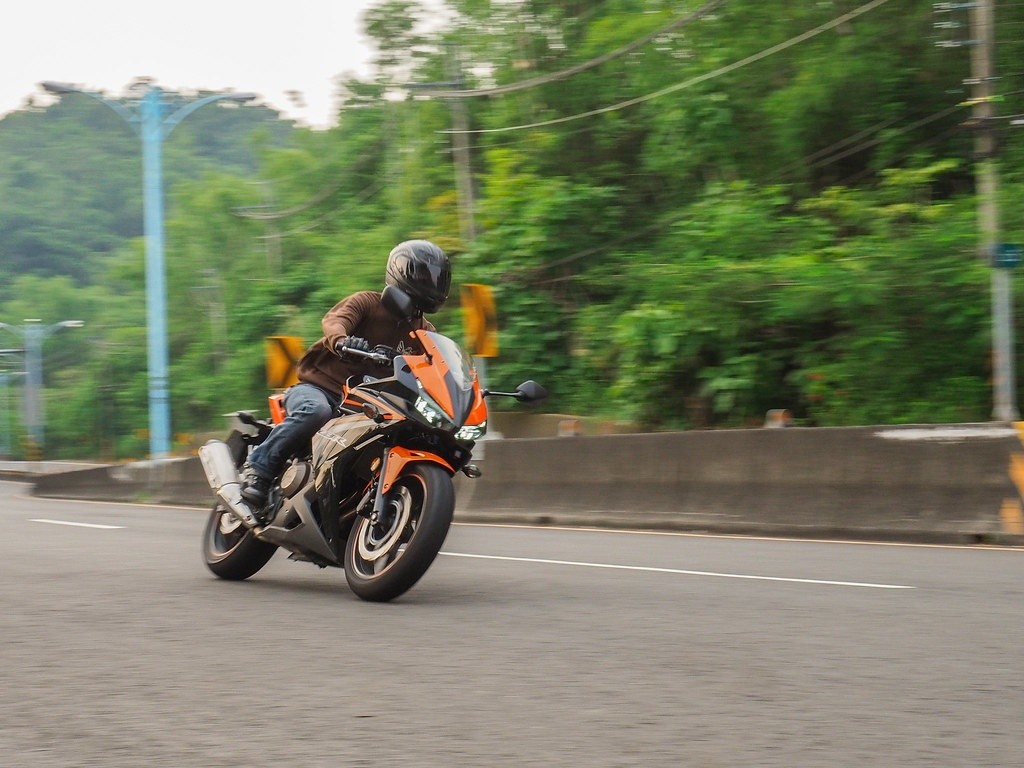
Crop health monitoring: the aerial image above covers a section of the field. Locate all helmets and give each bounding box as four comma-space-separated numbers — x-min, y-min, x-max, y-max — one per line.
384, 239, 452, 314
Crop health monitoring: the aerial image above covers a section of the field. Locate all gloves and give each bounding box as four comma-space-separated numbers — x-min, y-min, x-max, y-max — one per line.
343, 334, 369, 352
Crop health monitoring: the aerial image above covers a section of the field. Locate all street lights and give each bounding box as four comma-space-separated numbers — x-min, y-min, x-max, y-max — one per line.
0, 318, 84, 463
43, 81, 257, 457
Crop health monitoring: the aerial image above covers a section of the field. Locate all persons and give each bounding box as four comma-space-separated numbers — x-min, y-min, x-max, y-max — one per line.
235, 240, 452, 504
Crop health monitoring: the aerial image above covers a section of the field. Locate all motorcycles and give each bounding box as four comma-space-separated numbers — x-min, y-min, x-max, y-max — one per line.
197, 284, 547, 605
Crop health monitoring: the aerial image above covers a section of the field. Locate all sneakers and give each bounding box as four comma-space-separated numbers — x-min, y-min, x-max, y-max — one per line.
239, 460, 274, 508
